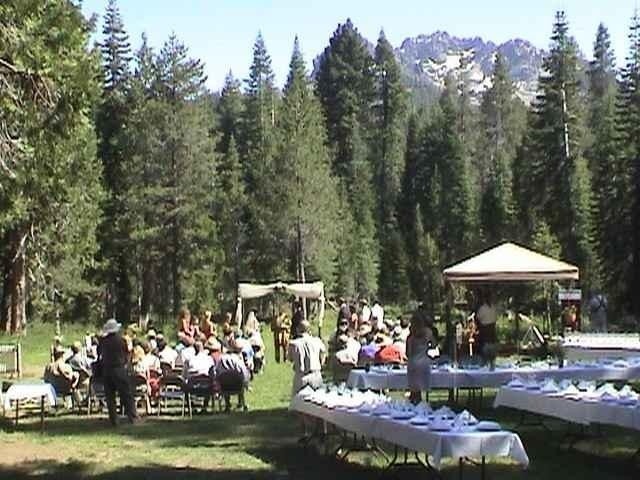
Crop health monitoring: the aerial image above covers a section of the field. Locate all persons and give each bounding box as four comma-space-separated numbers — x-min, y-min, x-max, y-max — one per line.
327, 287, 501, 407
288, 300, 305, 363
43, 307, 266, 425
288, 319, 328, 442
270, 309, 292, 364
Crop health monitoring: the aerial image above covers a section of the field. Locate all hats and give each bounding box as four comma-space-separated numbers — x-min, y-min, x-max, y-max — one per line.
176, 332, 221, 351
103, 319, 121, 332
144, 330, 156, 339
54, 340, 80, 354
296, 320, 310, 331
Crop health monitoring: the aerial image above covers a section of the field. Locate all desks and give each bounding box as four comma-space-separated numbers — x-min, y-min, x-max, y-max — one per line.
2, 382, 50, 432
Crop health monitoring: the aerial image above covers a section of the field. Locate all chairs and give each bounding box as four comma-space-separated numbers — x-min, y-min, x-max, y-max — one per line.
43, 345, 248, 421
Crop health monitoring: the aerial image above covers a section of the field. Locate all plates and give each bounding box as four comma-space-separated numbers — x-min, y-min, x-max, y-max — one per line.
304, 390, 502, 431
510, 382, 637, 406
